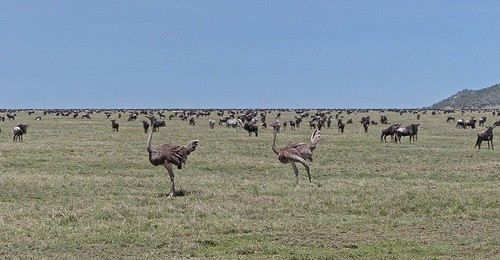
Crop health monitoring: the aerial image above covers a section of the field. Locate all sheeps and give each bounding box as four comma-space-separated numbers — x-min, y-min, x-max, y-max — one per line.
0, 108, 499, 151
